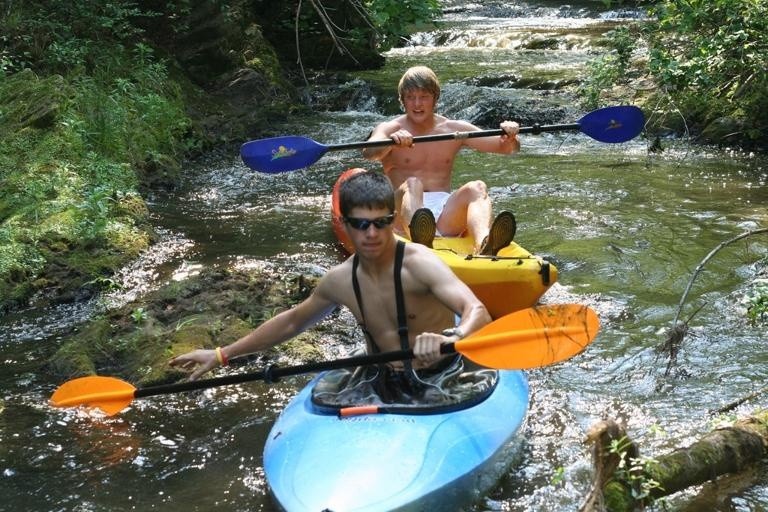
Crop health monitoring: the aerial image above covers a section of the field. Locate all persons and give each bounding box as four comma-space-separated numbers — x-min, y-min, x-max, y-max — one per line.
167, 172, 493, 383
362, 66, 521, 256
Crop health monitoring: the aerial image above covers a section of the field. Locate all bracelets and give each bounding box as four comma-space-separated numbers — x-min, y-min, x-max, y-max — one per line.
215, 347, 228, 366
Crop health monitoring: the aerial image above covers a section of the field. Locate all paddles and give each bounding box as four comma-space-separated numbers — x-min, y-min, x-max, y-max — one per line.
239, 105, 644, 172
49, 302, 599, 417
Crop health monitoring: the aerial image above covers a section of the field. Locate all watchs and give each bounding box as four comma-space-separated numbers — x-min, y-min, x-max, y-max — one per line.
441, 328, 464, 338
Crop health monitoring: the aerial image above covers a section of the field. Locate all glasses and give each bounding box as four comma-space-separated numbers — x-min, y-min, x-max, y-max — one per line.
343, 214, 394, 229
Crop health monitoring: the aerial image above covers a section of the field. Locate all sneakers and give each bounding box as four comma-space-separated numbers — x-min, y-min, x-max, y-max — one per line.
408, 208, 435, 248
479, 210, 516, 255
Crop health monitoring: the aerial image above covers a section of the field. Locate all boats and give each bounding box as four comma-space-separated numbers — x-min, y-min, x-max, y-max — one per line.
331, 167, 558, 319
261, 350, 528, 512
